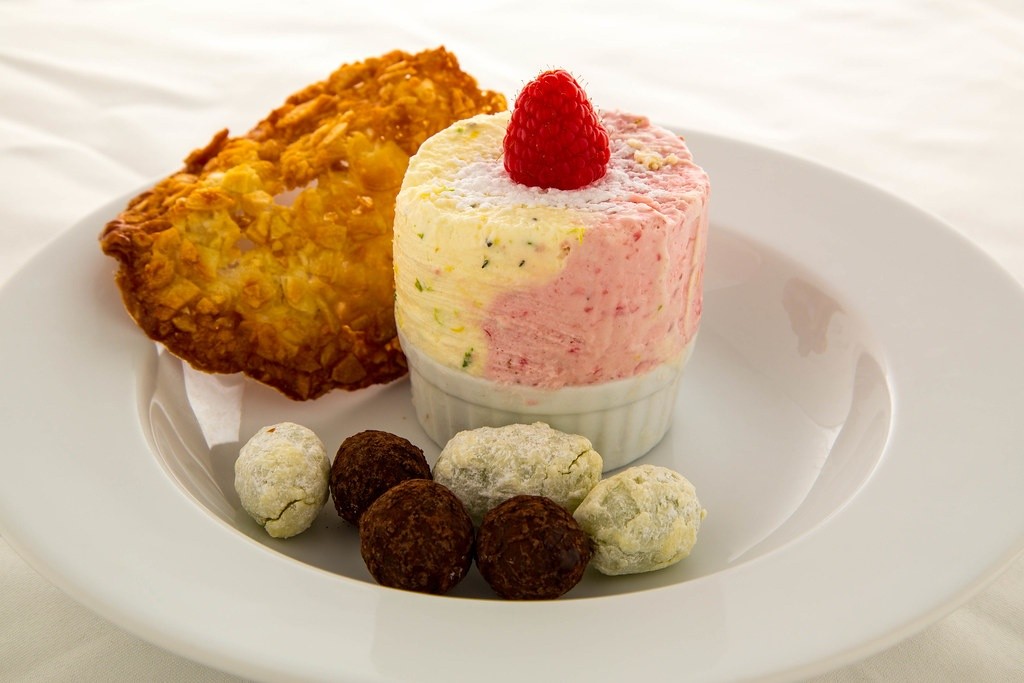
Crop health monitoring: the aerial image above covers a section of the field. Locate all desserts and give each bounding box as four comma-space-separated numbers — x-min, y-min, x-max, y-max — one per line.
392, 66, 711, 389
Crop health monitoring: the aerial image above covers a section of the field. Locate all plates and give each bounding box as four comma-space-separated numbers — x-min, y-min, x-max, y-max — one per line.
2, 123, 1023, 683
